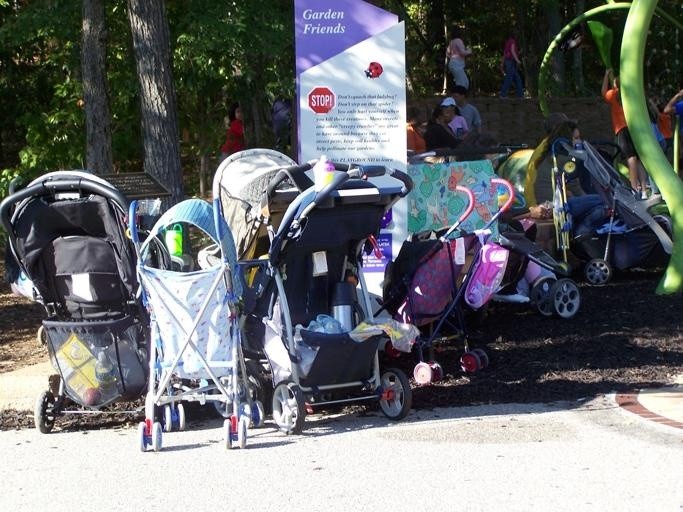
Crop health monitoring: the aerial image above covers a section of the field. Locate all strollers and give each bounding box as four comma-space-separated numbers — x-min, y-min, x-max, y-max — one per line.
129, 198, 269, 453
197, 149, 413, 434
551, 138, 672, 285
407, 147, 583, 318
1, 171, 195, 433
373, 178, 513, 385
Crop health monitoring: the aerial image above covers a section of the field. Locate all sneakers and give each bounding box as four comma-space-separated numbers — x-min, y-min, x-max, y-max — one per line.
641, 190, 648, 200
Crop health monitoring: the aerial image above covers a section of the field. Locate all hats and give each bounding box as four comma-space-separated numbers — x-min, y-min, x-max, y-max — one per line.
441, 97, 456, 106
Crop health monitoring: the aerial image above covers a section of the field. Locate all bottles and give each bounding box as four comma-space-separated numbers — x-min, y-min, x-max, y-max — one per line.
95, 352, 118, 403
164, 224, 182, 258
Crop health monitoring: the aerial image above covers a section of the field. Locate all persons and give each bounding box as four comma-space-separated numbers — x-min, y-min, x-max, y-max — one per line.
407, 108, 426, 153
446, 26, 472, 89
664, 79, 683, 137
450, 86, 481, 143
534, 123, 615, 243
220, 104, 245, 162
423, 106, 459, 150
653, 97, 675, 140
600, 67, 650, 199
499, 28, 525, 99
524, 112, 579, 210
272, 95, 292, 153
440, 98, 470, 141
